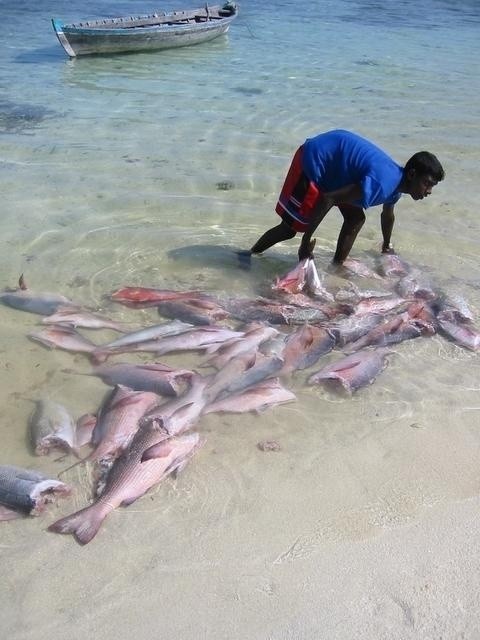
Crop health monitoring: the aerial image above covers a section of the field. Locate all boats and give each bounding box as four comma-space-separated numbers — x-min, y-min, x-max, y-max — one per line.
51, 0, 238, 56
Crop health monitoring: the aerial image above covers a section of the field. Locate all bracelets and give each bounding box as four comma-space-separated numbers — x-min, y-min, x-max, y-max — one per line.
384, 243, 393, 248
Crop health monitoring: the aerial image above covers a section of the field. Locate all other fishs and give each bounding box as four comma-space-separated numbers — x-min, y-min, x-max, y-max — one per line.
73, 239, 480, 471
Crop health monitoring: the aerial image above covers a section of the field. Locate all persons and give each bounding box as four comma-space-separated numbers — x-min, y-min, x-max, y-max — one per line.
235, 129, 445, 275
